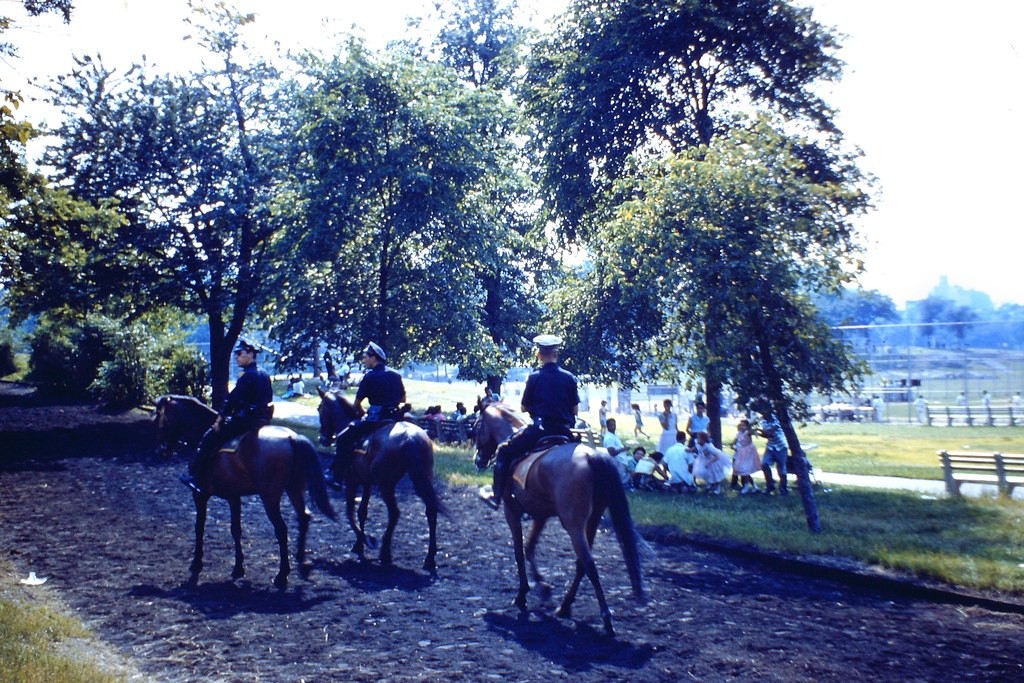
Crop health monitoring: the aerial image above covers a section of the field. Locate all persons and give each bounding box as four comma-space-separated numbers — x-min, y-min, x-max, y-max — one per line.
181, 340, 274, 487
598, 399, 790, 495
324, 347, 351, 388
475, 335, 580, 511
324, 341, 406, 490
287, 377, 305, 397
402, 386, 500, 435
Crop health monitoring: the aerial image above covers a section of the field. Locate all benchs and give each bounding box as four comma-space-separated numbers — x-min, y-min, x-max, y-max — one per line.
406, 416, 473, 442
936, 451, 1024, 495
816, 406, 877, 422
571, 429, 600, 448
925, 405, 1024, 427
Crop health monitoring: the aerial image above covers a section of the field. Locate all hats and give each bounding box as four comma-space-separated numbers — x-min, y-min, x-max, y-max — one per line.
234, 337, 259, 352
533, 334, 563, 349
363, 340, 386, 362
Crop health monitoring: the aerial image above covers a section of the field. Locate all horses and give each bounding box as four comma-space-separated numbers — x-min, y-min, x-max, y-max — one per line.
470, 395, 646, 637
148, 395, 336, 593
316, 385, 454, 570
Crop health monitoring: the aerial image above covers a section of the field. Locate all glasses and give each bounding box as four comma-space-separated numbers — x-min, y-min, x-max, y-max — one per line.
235, 351, 247, 356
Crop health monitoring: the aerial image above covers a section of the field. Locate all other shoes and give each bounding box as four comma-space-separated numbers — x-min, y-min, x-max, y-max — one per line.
324, 468, 342, 486
479, 490, 499, 509
646, 435, 650, 441
713, 488, 721, 494
181, 473, 202, 493
751, 484, 760, 493
764, 484, 776, 495
740, 484, 749, 494
779, 482, 789, 494
705, 483, 712, 490
